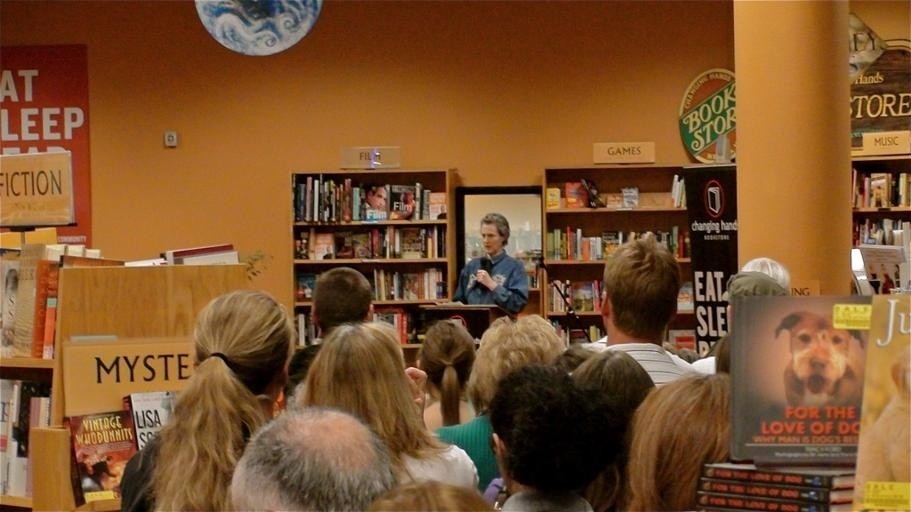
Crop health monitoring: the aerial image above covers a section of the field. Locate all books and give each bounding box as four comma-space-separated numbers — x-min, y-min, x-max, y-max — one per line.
854, 218, 911, 295
373, 268, 445, 301
547, 279, 603, 313
295, 225, 445, 259
0, 378, 50, 499
64, 410, 137, 508
852, 168, 910, 206
297, 311, 409, 344
729, 295, 874, 464
547, 224, 690, 259
670, 174, 686, 208
0, 242, 126, 358
854, 296, 911, 512
695, 462, 856, 512
122, 389, 181, 451
292, 173, 447, 222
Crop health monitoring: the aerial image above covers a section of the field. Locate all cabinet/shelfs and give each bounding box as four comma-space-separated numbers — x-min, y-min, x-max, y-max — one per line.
0, 262, 248, 511
539, 164, 696, 356
287, 168, 458, 369
850, 154, 911, 282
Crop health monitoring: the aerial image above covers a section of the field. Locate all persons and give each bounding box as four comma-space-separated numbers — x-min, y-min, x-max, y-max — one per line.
627, 374, 730, 512
231, 409, 394, 511
598, 239, 691, 389
451, 212, 528, 313
120, 290, 295, 511
296, 323, 479, 493
434, 314, 567, 490
691, 272, 791, 374
489, 364, 606, 511
416, 321, 478, 434
287, 268, 374, 385
570, 349, 657, 415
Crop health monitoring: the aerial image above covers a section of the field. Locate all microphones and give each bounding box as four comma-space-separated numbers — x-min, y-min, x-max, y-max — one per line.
473, 256, 488, 289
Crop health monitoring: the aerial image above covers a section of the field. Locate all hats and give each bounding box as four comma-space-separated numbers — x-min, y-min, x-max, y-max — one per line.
722, 271, 788, 304
